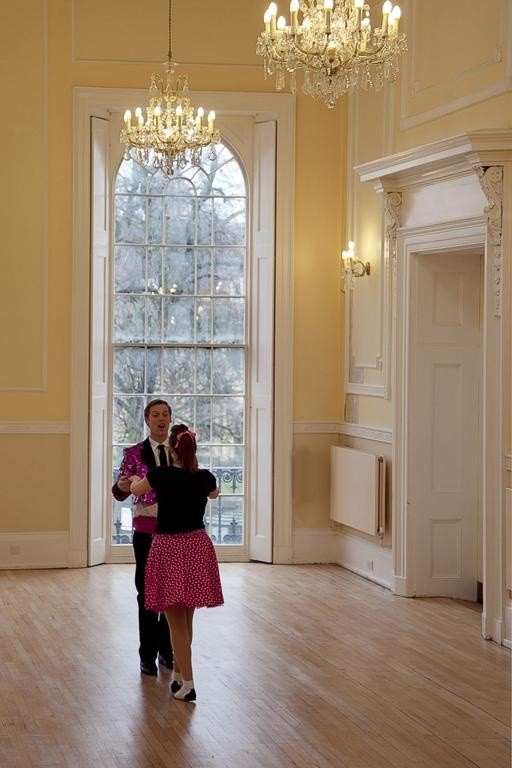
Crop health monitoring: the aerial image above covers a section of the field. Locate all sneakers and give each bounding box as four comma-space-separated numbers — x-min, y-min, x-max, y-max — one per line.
173, 686, 196, 702
169, 680, 182, 693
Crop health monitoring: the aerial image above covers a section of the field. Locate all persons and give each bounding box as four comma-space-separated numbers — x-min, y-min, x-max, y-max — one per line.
126, 422, 220, 702
112, 398, 177, 677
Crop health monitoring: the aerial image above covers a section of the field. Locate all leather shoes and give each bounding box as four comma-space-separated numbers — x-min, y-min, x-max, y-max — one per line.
159, 656, 173, 670
140, 661, 157, 676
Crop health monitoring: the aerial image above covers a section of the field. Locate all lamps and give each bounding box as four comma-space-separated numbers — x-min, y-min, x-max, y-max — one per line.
338, 242, 371, 294
114, 0, 222, 174
255, 0, 410, 108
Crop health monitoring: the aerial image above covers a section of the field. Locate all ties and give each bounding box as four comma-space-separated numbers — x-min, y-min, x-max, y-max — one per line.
157, 445, 168, 467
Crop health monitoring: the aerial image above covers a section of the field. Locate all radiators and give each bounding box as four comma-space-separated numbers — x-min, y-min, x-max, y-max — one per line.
330, 446, 386, 546
